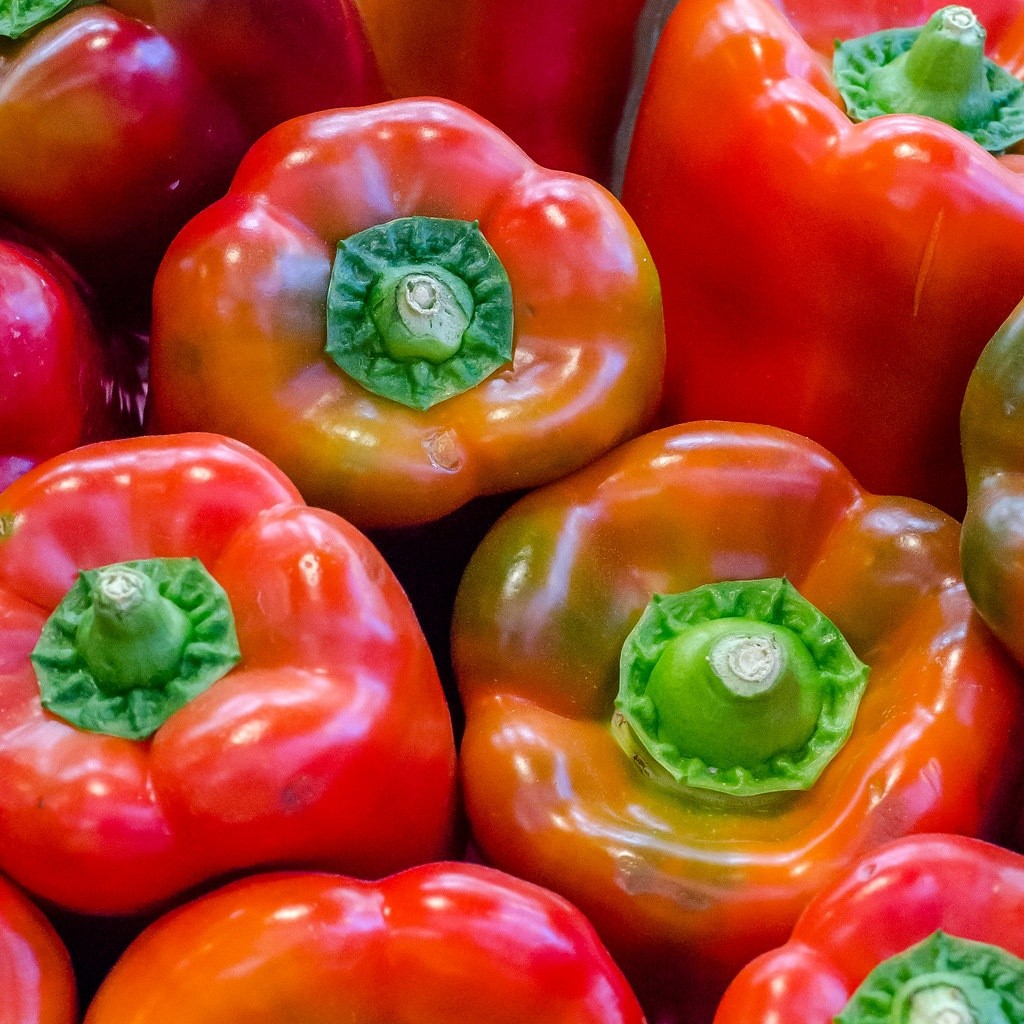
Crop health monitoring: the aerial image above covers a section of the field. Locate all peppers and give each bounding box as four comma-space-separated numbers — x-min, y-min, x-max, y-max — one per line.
0, 0, 1024, 1024
